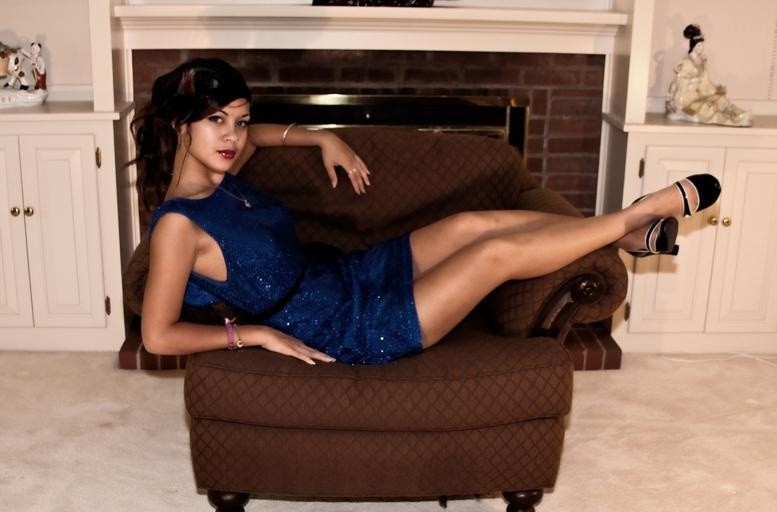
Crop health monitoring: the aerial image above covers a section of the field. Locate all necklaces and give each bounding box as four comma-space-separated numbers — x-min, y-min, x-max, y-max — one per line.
219, 176, 253, 212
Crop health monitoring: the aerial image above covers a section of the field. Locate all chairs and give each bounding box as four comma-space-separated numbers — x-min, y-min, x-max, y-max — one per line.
121, 124, 628, 511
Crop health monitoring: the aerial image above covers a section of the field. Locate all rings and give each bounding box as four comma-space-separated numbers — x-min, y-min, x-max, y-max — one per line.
346, 170, 353, 177
351, 167, 357, 174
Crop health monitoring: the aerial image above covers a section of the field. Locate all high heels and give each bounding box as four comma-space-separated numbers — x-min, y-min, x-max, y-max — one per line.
631, 174, 720, 218
620, 216, 679, 258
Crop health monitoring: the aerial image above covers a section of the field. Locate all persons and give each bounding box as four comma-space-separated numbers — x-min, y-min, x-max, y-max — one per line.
120, 53, 721, 374
18, 41, 50, 90
0, 42, 31, 90
662, 22, 752, 128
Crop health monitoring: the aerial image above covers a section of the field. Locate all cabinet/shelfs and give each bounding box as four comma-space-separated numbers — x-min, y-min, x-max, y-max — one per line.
606, 132, 776, 353
0, 118, 128, 351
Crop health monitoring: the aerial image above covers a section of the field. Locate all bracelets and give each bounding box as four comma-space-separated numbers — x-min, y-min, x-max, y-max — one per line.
278, 122, 297, 148
221, 315, 234, 353
230, 323, 246, 351
